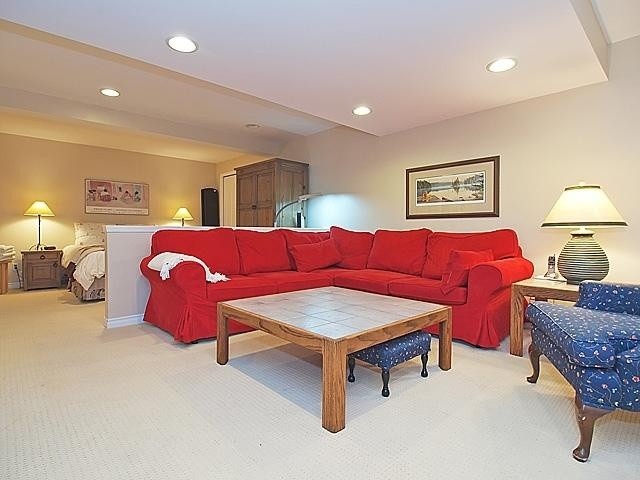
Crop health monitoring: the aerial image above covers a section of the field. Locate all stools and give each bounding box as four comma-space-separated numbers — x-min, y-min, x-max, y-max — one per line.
347, 329, 431, 397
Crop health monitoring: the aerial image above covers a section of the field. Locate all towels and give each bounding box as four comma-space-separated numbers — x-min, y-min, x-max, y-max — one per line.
149, 251, 231, 283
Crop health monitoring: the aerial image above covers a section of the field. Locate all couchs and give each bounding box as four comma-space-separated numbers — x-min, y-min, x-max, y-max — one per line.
138, 224, 535, 350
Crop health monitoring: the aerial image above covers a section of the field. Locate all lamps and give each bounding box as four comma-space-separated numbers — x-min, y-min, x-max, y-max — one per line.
172, 208, 194, 227
272, 192, 322, 226
539, 184, 630, 285
23, 200, 56, 249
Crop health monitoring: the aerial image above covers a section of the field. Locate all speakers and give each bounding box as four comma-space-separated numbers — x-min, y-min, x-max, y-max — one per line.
201, 188, 220, 226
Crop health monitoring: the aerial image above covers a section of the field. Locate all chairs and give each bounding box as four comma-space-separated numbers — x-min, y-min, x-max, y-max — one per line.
525, 279, 640, 463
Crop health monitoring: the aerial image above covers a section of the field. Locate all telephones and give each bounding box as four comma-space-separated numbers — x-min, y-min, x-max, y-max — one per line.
544, 254, 558, 279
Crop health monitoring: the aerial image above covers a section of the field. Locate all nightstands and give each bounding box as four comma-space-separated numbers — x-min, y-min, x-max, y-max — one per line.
21, 249, 64, 291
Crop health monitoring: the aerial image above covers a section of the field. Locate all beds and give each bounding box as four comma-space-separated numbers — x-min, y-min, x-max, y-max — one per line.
60, 245, 106, 302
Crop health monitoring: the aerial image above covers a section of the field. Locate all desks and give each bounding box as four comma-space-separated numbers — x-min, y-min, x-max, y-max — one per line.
215, 286, 454, 434
0, 261, 12, 294
509, 275, 581, 358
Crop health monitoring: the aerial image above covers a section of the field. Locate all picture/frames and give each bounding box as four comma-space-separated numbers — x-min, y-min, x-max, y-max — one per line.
85, 179, 149, 216
405, 155, 501, 220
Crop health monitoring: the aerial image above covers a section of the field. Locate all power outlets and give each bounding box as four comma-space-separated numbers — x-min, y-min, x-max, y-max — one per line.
14, 264, 18, 271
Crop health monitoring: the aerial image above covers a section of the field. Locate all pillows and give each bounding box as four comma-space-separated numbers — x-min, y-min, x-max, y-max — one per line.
287, 237, 342, 274
438, 248, 496, 294
73, 222, 106, 247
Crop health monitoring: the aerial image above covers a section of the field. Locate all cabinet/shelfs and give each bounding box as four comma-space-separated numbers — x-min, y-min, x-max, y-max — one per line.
234, 157, 309, 227
